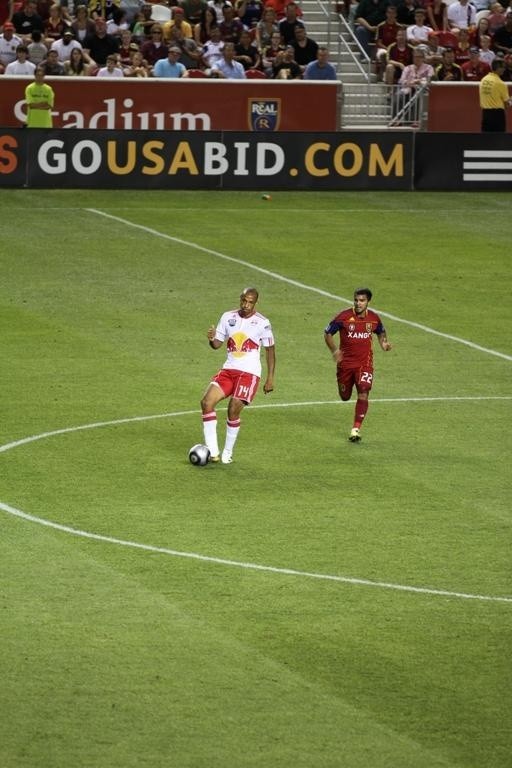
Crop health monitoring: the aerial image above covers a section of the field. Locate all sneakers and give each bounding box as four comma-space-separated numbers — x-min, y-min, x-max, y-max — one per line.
348, 431, 361, 442
211, 454, 232, 464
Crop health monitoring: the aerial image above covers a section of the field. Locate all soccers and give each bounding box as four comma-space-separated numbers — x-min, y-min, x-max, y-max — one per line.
188, 445, 210, 466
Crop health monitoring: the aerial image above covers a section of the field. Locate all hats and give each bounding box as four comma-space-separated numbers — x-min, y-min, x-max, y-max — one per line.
2, 7, 185, 55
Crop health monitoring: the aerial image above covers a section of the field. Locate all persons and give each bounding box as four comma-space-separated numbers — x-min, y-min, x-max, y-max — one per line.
200, 287, 275, 464
479, 57, 509, 132
336, 0, 512, 127
1, 0, 334, 80
324, 287, 393, 443
25, 65, 55, 128
